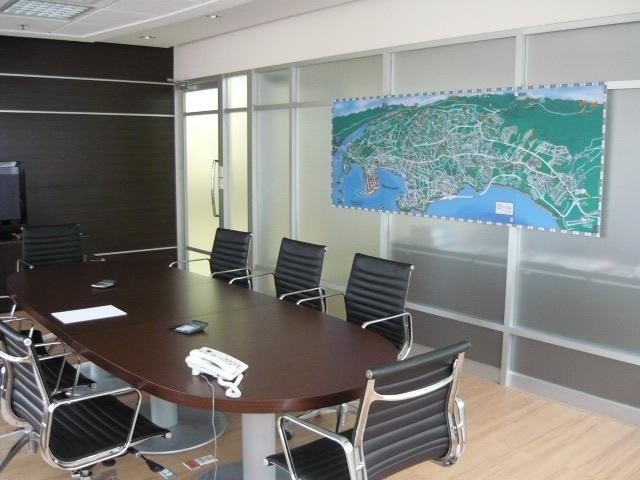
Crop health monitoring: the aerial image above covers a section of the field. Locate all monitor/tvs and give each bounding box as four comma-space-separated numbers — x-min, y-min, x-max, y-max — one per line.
0, 162, 26, 241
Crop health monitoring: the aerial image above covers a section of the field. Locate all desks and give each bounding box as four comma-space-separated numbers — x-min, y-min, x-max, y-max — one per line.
8, 258, 401, 479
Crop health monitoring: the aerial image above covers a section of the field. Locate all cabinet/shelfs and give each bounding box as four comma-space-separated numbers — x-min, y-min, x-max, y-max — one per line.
0, 237, 28, 311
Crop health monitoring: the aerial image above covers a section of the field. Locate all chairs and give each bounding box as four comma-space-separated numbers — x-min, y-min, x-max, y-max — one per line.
15, 221, 88, 276
228, 236, 330, 315
0, 291, 178, 480
280, 252, 415, 440
260, 338, 474, 480
166, 227, 253, 291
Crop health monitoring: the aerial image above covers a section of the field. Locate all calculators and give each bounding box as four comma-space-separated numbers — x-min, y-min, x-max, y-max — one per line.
174, 320, 208, 334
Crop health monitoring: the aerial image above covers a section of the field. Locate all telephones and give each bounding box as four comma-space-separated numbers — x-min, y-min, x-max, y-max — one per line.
185, 346, 249, 381
